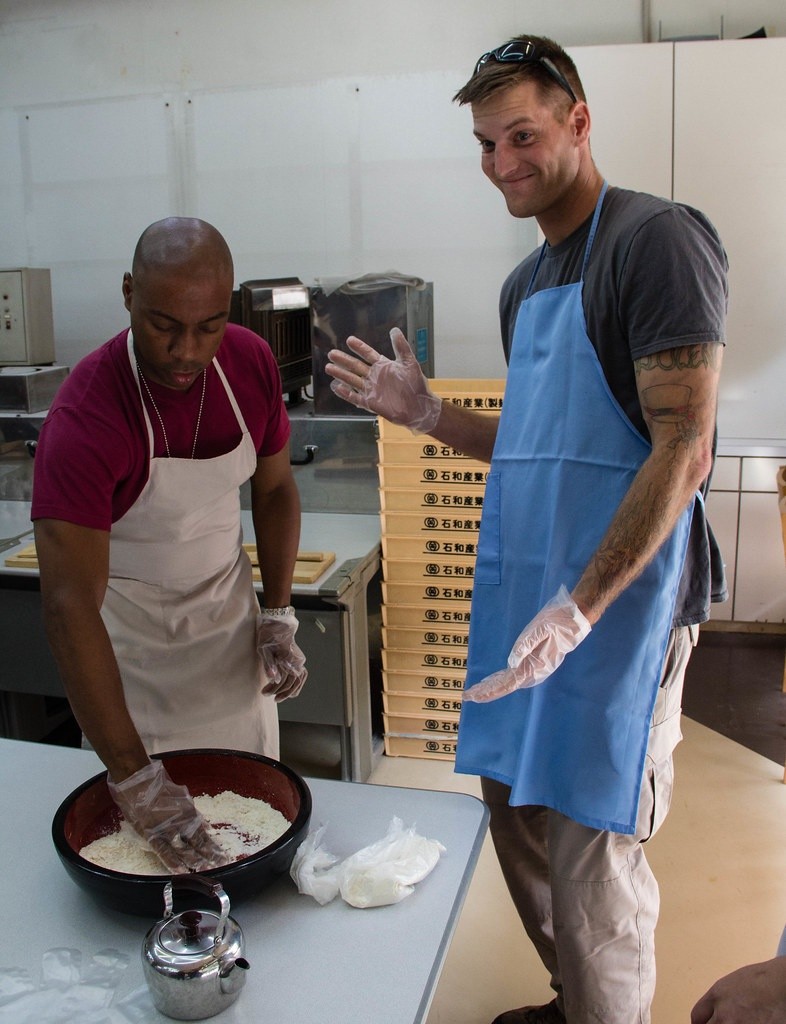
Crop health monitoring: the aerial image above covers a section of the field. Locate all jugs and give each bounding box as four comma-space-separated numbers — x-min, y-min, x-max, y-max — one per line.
143, 876, 252, 1023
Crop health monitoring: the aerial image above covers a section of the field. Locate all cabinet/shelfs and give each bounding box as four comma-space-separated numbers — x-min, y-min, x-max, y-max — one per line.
0, 268, 57, 367
703, 443, 786, 640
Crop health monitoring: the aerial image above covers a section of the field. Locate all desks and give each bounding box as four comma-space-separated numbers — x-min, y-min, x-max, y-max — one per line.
0, 737, 492, 1023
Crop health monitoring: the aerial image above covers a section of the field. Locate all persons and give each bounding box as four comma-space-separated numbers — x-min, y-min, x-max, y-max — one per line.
319, 28, 731, 1024
692, 909, 786, 1024
31, 218, 312, 878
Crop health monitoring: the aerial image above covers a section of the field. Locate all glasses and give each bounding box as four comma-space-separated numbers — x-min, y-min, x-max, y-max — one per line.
471, 39, 577, 104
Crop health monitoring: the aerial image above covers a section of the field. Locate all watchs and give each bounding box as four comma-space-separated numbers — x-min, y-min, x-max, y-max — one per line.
260, 603, 297, 619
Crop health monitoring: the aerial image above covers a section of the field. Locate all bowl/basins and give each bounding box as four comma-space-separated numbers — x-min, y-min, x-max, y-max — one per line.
51, 746, 311, 911
